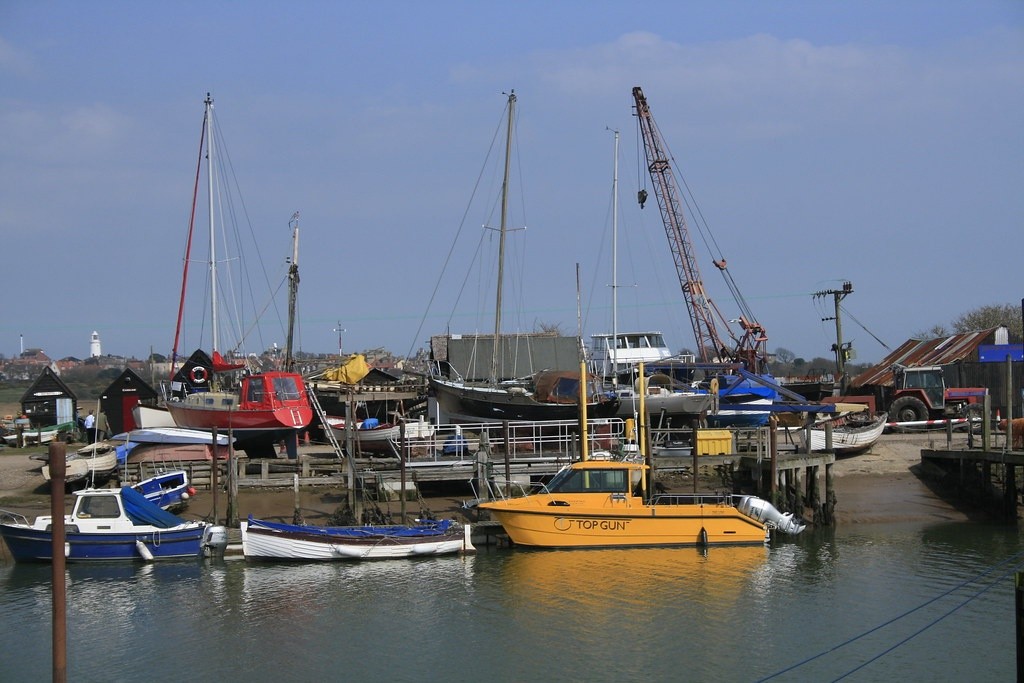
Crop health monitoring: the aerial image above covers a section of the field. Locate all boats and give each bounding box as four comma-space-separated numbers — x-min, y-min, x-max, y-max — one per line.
1, 85, 844, 567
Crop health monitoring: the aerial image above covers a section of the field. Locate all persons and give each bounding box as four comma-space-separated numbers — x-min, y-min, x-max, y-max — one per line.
84, 409, 96, 444
97, 408, 110, 442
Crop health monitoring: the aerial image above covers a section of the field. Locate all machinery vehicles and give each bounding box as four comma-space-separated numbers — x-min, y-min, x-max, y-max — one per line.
776, 362, 989, 434
623, 86, 785, 391
